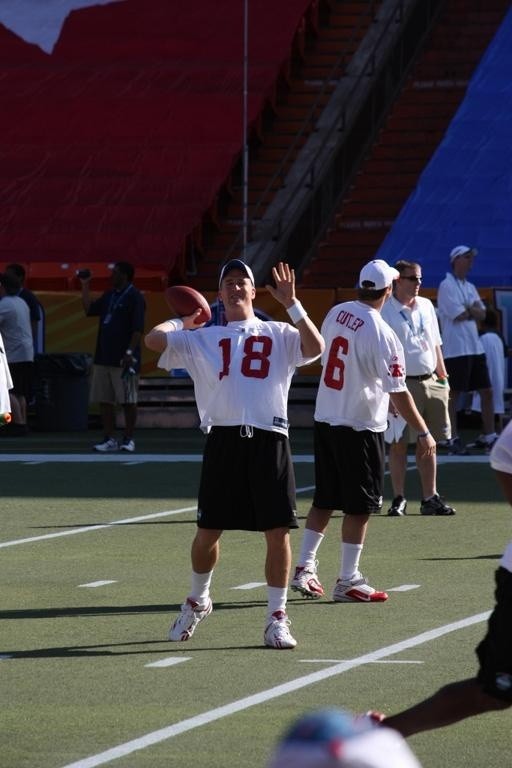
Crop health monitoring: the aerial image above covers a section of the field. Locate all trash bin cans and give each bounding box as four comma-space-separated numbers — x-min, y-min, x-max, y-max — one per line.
30, 353, 94, 432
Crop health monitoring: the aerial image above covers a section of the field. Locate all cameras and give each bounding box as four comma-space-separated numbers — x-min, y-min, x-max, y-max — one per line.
75, 269, 90, 278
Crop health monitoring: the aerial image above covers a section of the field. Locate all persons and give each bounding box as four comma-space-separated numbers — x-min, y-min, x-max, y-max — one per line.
78, 259, 147, 452
143, 258, 328, 649
436, 245, 506, 456
379, 259, 456, 517
290, 260, 434, 605
474, 421, 512, 700
0, 263, 44, 426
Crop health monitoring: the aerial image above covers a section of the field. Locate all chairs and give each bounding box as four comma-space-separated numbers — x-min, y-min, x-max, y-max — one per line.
354, 0, 512, 290
1, 0, 318, 290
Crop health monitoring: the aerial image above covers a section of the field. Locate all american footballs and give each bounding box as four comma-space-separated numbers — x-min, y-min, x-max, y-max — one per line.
166, 285, 212, 324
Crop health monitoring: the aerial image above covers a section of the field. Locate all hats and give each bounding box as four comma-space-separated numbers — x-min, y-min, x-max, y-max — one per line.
218, 258, 254, 289
358, 259, 400, 291
449, 245, 478, 263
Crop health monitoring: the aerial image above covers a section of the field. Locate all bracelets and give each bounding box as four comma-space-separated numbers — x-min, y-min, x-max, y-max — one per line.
287, 301, 308, 325
419, 431, 429, 437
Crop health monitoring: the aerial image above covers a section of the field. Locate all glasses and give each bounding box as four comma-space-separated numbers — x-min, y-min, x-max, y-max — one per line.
401, 275, 423, 281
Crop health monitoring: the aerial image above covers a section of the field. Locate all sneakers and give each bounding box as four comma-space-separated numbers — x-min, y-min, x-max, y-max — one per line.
93, 435, 118, 452
120, 438, 136, 452
263, 610, 297, 648
420, 494, 456, 515
291, 564, 324, 599
332, 578, 388, 602
447, 439, 467, 456
388, 495, 407, 515
169, 597, 213, 642
